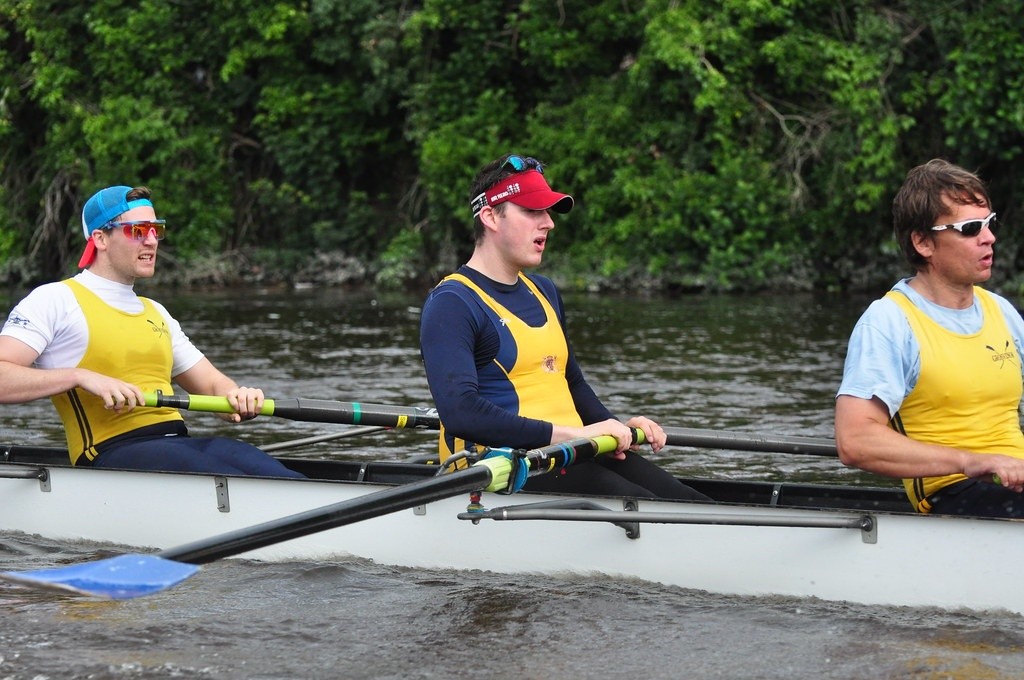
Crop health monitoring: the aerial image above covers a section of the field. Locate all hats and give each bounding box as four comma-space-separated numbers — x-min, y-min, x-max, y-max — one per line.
471, 169, 574, 217
78, 186, 153, 268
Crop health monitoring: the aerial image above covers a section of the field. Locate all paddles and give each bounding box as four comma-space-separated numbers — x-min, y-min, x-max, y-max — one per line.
0, 426, 635, 600
108, 387, 441, 436
635, 427, 840, 460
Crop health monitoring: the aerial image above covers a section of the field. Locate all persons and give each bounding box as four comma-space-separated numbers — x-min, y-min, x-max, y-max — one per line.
418, 151, 714, 504
834, 158, 1024, 520
0, 186, 309, 480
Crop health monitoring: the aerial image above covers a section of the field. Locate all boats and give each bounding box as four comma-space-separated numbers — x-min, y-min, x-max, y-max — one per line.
1, 445, 1024, 615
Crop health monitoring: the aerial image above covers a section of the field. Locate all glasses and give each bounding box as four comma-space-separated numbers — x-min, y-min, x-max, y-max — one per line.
483, 155, 544, 187
100, 220, 167, 240
929, 212, 998, 236
